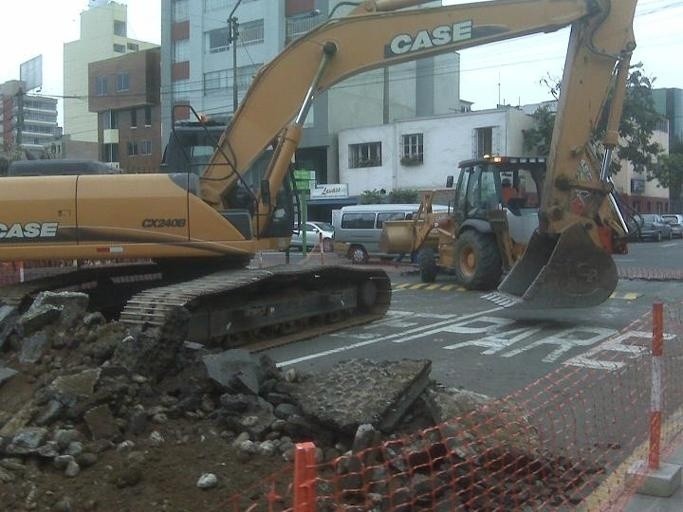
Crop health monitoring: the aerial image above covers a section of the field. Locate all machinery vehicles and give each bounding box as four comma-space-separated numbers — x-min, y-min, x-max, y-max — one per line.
378, 154, 547, 293
0, 0, 638, 362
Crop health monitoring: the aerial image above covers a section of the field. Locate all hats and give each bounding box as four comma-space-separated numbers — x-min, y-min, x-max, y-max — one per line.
502, 179, 510, 185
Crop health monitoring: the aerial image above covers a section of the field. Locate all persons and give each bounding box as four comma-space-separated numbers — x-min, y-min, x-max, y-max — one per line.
501, 177, 515, 206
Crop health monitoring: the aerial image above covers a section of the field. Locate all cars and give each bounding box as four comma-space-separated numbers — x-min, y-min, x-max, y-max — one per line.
628, 213, 673, 242
279, 220, 335, 253
661, 213, 683, 238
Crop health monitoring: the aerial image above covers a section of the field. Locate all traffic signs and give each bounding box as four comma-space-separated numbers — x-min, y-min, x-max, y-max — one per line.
288, 180, 316, 191
291, 170, 316, 180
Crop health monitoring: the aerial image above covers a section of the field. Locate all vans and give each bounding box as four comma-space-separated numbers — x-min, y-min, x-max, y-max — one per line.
334, 203, 454, 265
